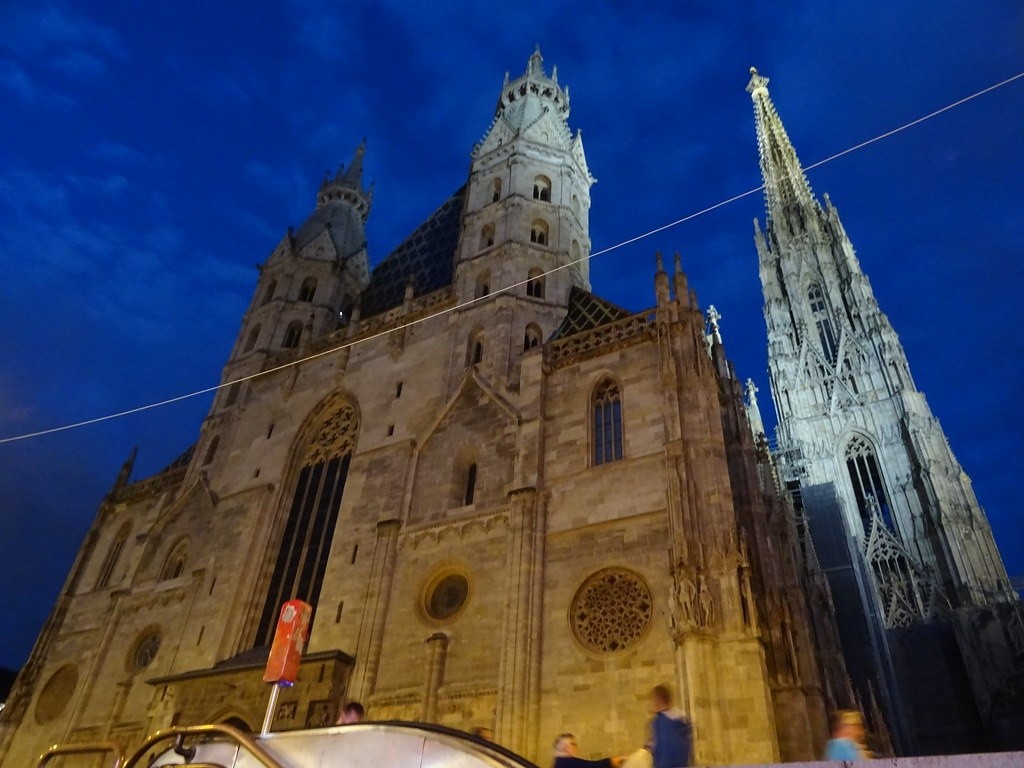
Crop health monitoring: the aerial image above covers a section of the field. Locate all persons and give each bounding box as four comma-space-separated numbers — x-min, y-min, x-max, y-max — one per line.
821, 711, 871, 760
647, 685, 690, 768
334, 702, 365, 724
552, 733, 627, 768
